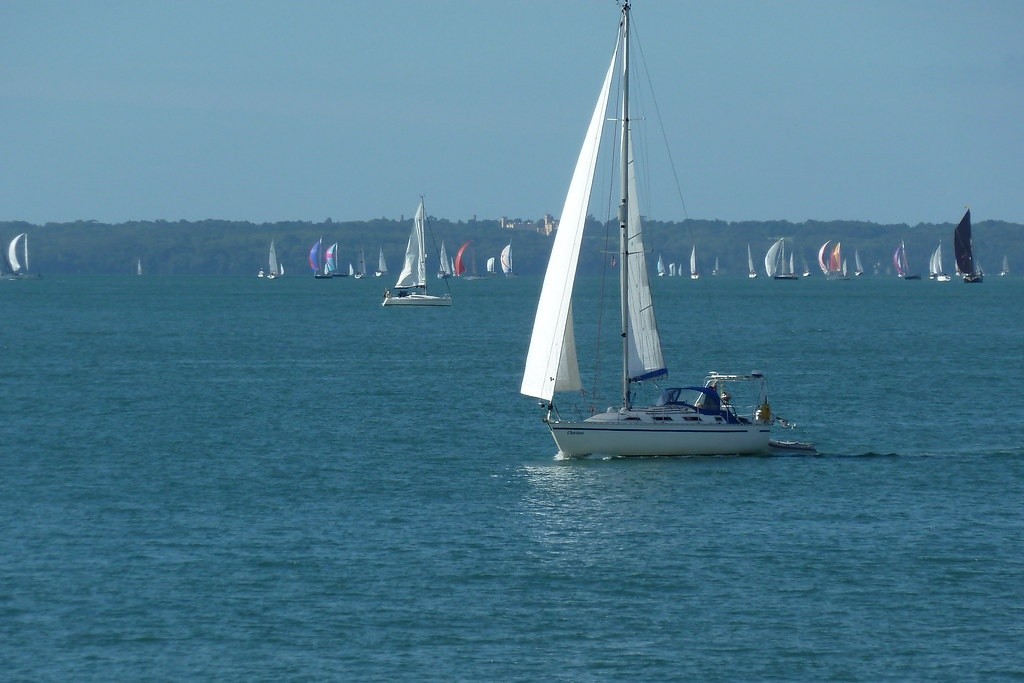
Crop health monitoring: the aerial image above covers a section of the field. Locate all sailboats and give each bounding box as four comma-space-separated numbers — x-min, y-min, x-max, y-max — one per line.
500, 242, 519, 279
997, 255, 1009, 276
819, 239, 864, 277
451, 257, 456, 277
934, 240, 951, 282
436, 240, 451, 279
712, 257, 719, 276
765, 237, 799, 280
669, 263, 675, 277
8, 233, 43, 280
350, 264, 354, 276
955, 207, 983, 283
929, 253, 934, 279
455, 240, 489, 280
380, 195, 454, 307
657, 255, 666, 277
309, 236, 333, 280
894, 239, 922, 280
519, 0, 771, 458
748, 244, 757, 279
355, 245, 366, 279
897, 256, 903, 278
375, 248, 388, 277
266, 240, 279, 280
679, 264, 682, 276
485, 257, 497, 275
690, 245, 699, 280
324, 243, 348, 278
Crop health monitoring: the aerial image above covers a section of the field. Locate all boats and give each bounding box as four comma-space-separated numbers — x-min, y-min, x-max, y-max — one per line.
802, 270, 811, 277
769, 438, 818, 456
257, 268, 264, 278
